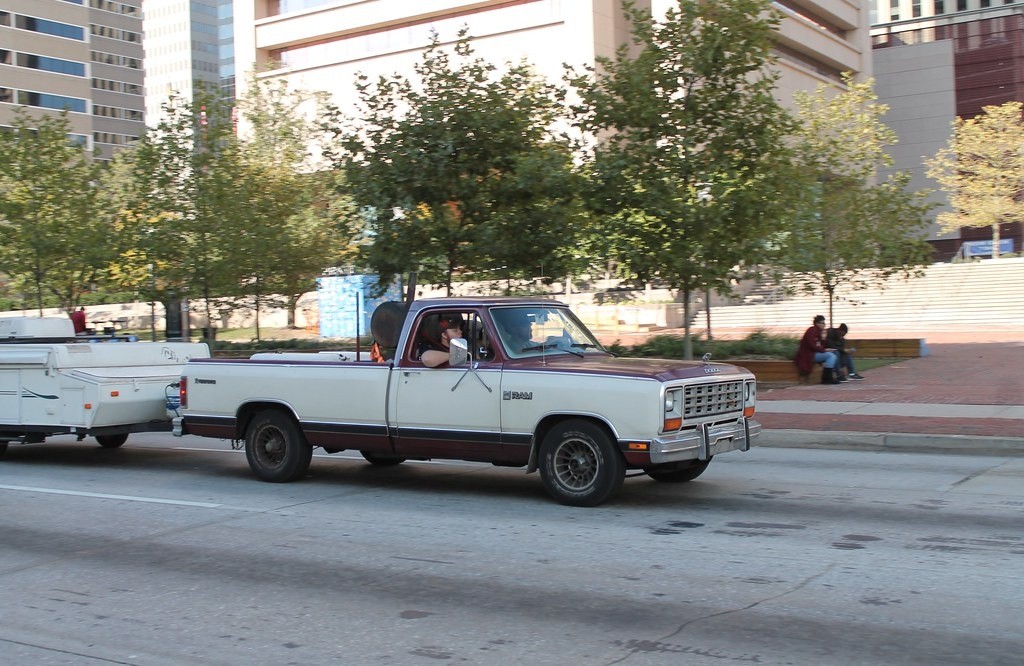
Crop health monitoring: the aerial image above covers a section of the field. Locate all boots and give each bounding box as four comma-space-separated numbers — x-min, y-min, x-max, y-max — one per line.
821, 368, 840, 384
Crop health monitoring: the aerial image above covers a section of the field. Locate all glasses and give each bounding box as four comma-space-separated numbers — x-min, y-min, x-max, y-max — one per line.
818, 323, 824, 324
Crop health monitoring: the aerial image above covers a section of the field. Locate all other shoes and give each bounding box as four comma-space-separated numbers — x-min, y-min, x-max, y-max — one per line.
848, 373, 866, 380
837, 377, 849, 382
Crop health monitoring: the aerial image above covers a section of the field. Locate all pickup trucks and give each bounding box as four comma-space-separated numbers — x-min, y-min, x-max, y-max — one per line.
172, 295, 764, 508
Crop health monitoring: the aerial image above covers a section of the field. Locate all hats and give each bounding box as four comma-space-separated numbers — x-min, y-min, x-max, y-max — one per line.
840, 324, 848, 333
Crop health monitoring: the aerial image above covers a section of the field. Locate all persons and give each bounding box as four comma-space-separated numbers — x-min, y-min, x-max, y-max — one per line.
68, 306, 86, 334
509, 314, 570, 354
419, 318, 480, 368
794, 313, 864, 385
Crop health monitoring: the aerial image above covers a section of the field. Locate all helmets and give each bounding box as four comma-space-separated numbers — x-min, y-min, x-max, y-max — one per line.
435, 314, 465, 338
